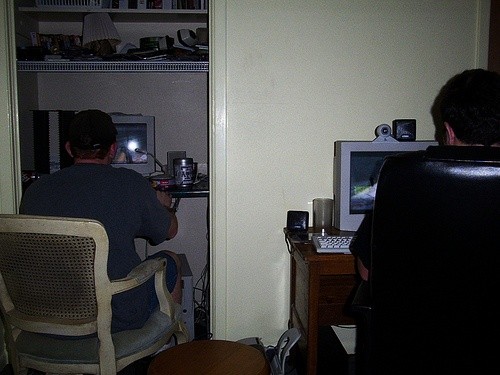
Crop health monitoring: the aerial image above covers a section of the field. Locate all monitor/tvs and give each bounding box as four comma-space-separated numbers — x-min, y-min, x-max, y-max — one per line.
332, 140, 439, 231
109, 116, 157, 175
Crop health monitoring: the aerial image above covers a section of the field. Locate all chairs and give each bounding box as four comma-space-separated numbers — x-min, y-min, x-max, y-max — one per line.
356, 154, 500, 375
0, 214, 190, 375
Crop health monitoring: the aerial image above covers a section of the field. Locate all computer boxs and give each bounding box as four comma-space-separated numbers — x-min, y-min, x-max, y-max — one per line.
152, 254, 194, 354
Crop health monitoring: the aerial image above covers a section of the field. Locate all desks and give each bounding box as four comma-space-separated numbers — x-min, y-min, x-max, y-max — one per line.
284, 226, 361, 375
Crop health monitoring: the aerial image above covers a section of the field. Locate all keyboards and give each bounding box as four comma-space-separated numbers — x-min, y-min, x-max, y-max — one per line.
313, 235, 354, 254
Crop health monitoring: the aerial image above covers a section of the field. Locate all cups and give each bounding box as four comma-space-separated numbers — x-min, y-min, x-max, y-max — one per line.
172, 157, 198, 191
311, 198, 334, 233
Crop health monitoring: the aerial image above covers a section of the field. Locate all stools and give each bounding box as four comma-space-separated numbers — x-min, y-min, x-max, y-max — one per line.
148, 340, 272, 375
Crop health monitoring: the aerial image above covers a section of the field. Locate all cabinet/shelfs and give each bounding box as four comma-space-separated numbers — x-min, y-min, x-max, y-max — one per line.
8, 0, 212, 333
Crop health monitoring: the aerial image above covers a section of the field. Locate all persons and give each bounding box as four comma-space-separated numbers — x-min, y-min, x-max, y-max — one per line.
345, 69, 500, 358
19, 108, 182, 340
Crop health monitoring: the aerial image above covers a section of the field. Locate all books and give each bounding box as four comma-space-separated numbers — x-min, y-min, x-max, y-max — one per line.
29, 32, 82, 53
34, 0, 208, 9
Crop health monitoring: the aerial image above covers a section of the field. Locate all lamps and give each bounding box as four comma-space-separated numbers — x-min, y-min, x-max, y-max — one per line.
82, 13, 122, 58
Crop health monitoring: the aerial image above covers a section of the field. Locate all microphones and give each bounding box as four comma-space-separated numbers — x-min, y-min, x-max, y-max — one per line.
135, 148, 167, 176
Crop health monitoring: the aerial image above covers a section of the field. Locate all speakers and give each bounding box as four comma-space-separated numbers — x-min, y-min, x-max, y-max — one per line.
286, 211, 309, 231
392, 119, 416, 142
